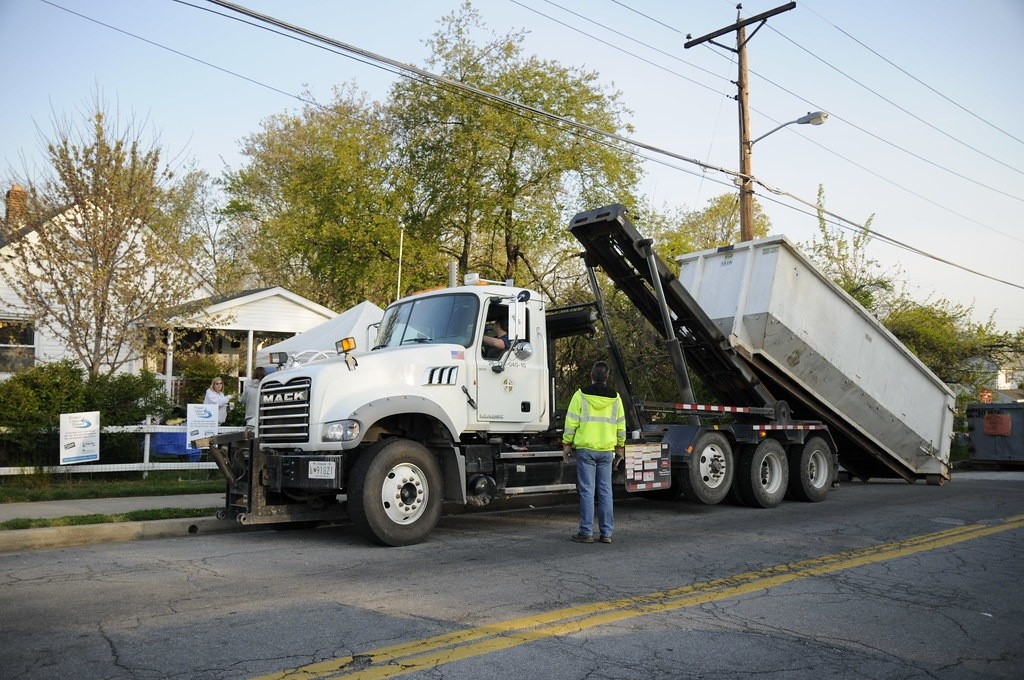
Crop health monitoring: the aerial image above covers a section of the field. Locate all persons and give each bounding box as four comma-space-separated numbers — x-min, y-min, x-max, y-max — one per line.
562, 361, 626, 543
483, 316, 510, 351
204, 377, 236, 426
238, 367, 266, 426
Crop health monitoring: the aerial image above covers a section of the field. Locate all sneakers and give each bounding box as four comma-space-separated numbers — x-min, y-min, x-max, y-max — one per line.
570, 532, 594, 542
597, 535, 612, 542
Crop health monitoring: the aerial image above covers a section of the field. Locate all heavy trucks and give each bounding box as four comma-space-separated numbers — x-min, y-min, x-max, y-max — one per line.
255, 202, 957, 548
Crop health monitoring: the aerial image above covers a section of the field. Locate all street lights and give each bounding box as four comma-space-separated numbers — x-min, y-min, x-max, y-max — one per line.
739, 112, 831, 244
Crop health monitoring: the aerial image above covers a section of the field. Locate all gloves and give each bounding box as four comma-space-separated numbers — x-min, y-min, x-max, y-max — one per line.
562, 443, 576, 463
614, 446, 625, 467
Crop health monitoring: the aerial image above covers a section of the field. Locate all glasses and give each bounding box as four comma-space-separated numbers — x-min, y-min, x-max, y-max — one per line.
216, 382, 223, 385
